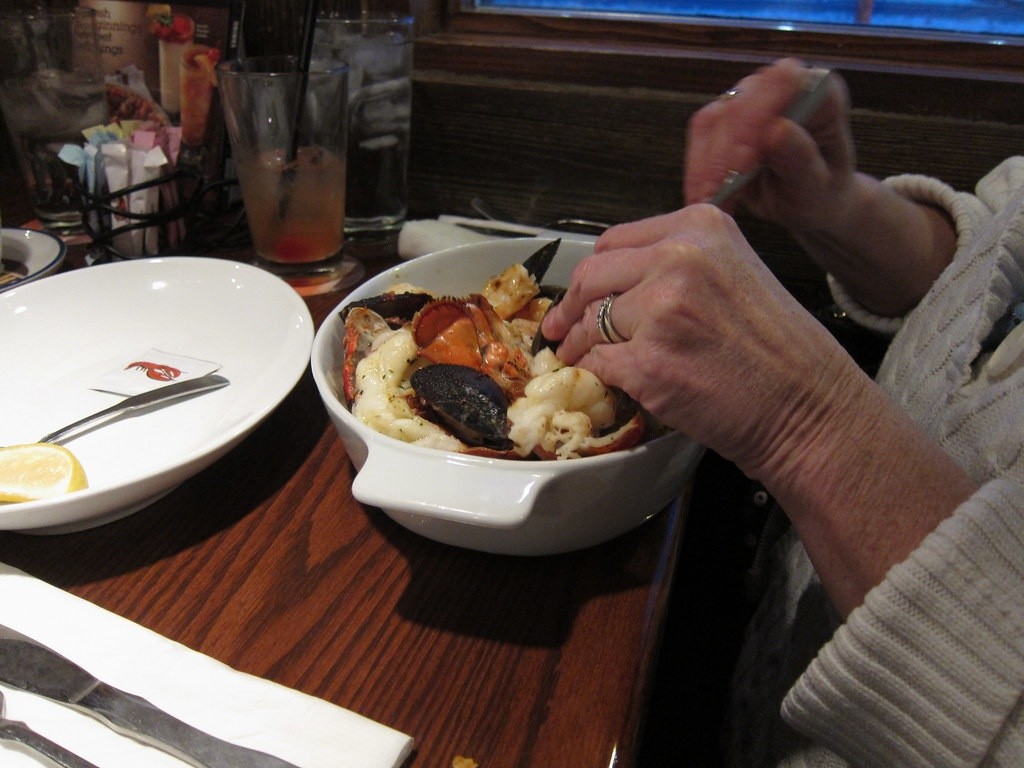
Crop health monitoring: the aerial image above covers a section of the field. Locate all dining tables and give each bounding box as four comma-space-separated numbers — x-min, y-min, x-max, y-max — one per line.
0, 216, 693, 768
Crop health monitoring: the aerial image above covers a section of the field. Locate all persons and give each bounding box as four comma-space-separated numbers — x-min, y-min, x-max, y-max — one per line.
538, 57, 1024, 768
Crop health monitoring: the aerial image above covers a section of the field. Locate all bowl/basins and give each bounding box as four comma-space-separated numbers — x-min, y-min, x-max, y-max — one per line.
311, 238, 707, 554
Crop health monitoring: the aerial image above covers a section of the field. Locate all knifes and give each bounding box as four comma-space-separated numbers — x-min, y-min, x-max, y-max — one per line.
0, 624, 301, 768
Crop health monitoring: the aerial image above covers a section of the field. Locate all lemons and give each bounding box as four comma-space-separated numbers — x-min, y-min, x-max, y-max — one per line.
0, 442, 89, 503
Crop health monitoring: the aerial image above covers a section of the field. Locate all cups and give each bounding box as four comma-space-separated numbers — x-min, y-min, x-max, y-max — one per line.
216, 59, 350, 275
1, 0, 113, 234
177, 44, 219, 147
312, 12, 415, 232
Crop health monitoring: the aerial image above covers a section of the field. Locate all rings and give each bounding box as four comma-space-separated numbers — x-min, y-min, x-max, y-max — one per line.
595, 293, 628, 345
719, 88, 743, 99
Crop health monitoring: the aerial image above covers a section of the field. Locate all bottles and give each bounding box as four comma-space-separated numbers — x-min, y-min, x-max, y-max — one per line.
159, 13, 197, 116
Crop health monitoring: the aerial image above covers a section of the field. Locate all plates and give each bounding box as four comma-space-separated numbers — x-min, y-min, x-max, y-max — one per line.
0, 227, 68, 294
0, 257, 315, 535
